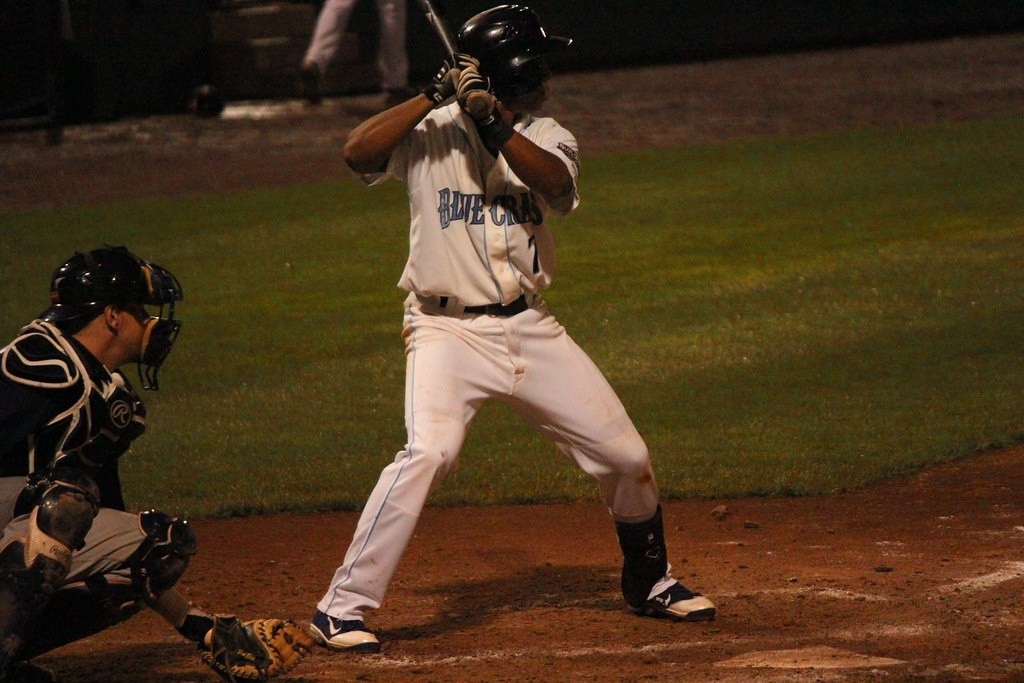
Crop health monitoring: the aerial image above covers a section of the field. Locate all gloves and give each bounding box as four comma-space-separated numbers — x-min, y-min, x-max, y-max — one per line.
421, 54, 480, 106
449, 66, 500, 129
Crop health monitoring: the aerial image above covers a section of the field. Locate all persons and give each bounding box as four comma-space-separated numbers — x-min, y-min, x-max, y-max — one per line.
0, 244, 314, 683
307, 2, 717, 654
299, 1, 423, 101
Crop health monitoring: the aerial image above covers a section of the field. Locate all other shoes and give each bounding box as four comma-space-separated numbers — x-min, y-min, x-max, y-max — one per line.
384, 88, 417, 105
302, 63, 323, 105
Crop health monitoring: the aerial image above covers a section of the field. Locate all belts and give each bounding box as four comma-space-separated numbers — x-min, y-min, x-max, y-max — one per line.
439, 294, 528, 317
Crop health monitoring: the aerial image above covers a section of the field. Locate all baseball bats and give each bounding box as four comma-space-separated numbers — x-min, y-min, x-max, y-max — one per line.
410, 0, 494, 119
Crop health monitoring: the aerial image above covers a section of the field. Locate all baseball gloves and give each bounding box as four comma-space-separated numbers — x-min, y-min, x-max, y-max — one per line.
196, 613, 320, 683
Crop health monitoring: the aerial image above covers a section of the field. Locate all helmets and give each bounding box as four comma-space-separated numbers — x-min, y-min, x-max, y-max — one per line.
459, 4, 572, 96
37, 247, 145, 322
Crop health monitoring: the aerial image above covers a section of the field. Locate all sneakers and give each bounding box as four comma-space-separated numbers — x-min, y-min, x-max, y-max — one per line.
628, 562, 716, 621
308, 610, 381, 654
